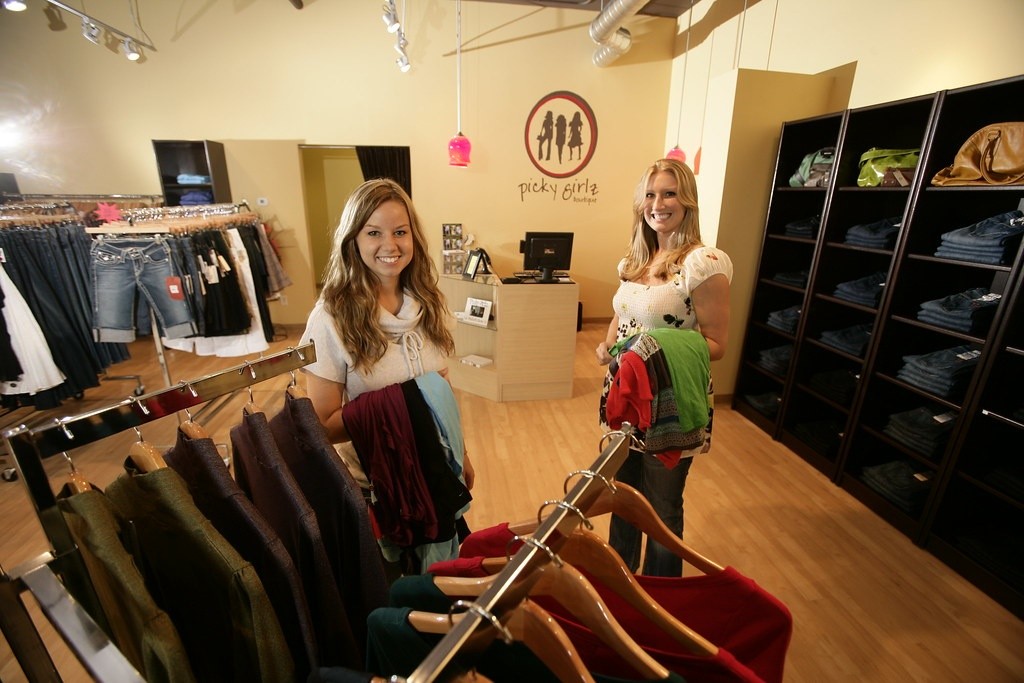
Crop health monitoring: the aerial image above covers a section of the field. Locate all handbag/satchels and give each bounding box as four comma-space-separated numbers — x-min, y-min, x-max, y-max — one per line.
857, 147, 921, 187
931, 122, 1024, 186
789, 147, 853, 188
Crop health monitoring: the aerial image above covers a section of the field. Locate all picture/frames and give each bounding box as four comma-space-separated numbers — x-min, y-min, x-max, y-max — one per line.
463, 295, 492, 327
461, 250, 482, 280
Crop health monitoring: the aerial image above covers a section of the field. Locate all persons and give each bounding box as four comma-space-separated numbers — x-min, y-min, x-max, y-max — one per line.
297, 180, 475, 580
596, 158, 733, 578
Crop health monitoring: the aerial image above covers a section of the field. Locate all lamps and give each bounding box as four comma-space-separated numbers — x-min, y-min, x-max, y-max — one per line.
1, 0, 27, 11
396, 58, 411, 72
448, 0, 471, 168
79, 17, 101, 47
122, 38, 140, 61
394, 37, 409, 55
382, 10, 400, 33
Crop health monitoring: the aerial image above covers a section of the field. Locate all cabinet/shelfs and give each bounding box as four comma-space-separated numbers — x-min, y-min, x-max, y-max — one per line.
729, 74, 1024, 620
151, 138, 232, 207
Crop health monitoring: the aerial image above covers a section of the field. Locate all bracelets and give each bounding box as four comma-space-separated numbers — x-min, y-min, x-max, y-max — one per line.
464, 451, 467, 455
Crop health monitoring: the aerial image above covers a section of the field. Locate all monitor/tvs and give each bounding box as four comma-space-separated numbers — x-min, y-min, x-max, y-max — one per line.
524, 230, 575, 284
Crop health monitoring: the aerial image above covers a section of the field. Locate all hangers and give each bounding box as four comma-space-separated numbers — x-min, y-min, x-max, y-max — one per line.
284, 346, 307, 401
178, 379, 210, 440
407, 535, 594, 683
244, 360, 262, 414
372, 600, 512, 683
484, 468, 718, 657
54, 418, 91, 495
1, 193, 259, 244
434, 501, 670, 681
508, 431, 727, 574
127, 396, 168, 473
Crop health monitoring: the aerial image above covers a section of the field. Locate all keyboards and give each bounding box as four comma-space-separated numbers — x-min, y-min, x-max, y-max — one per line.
513, 270, 571, 278
